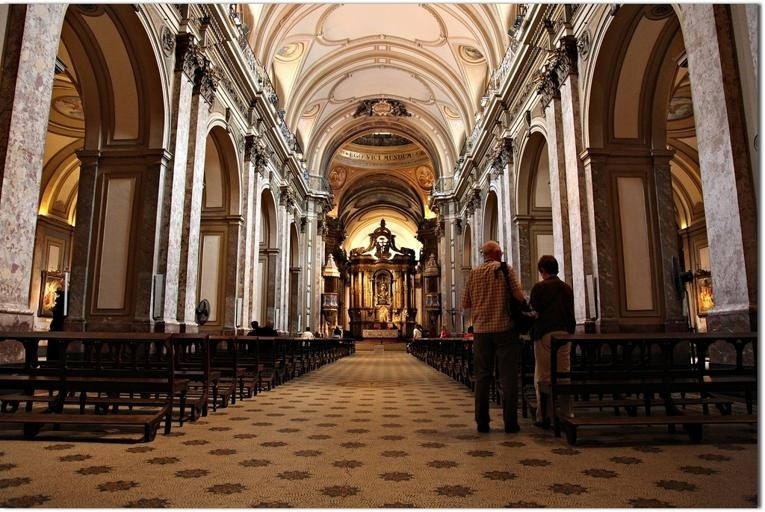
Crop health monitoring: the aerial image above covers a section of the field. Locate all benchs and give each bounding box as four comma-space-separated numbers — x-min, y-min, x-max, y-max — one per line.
0, 332, 357, 443
409, 332, 759, 446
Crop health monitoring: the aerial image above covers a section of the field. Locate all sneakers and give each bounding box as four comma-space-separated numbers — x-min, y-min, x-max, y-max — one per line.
477, 422, 521, 433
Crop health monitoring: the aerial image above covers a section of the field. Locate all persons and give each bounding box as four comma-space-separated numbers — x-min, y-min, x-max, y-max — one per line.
461, 240, 532, 433
528, 253, 577, 429
377, 283, 388, 304
247, 320, 342, 339
412, 324, 475, 350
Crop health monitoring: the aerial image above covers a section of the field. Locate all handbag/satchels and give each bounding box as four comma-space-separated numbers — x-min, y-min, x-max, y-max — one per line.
500, 262, 536, 337
